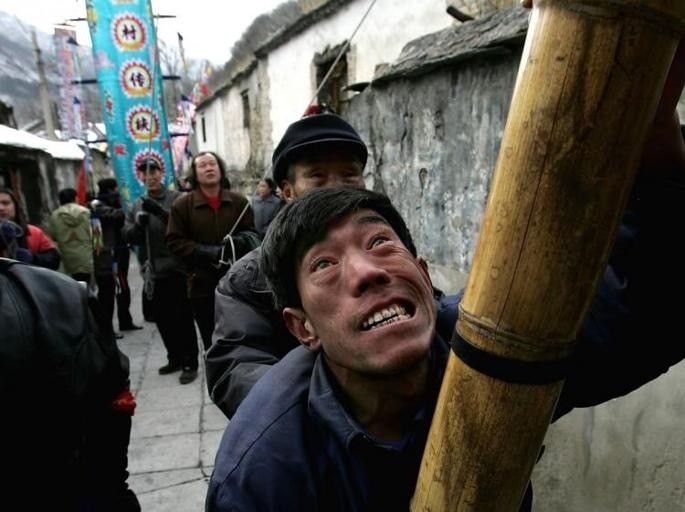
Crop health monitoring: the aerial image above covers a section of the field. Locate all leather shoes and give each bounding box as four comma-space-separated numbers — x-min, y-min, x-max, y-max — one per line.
114, 331, 124, 340
118, 323, 144, 331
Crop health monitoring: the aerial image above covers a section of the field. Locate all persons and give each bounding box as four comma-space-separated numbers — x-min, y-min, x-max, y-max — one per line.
0, 218, 140, 511
166, 151, 260, 353
166, 176, 198, 201
252, 177, 282, 240
1, 185, 62, 270
204, 114, 447, 420
125, 157, 199, 383
44, 178, 143, 340
201, 43, 685, 512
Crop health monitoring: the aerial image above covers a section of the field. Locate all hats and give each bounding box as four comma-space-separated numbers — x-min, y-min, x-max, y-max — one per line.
271, 113, 369, 187
137, 157, 162, 171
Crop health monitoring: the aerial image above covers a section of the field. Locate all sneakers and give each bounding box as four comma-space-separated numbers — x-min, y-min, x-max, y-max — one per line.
179, 369, 198, 385
158, 362, 183, 375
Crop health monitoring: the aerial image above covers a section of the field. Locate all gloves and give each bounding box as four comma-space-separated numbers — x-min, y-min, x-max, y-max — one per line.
134, 210, 151, 227
140, 195, 168, 220
